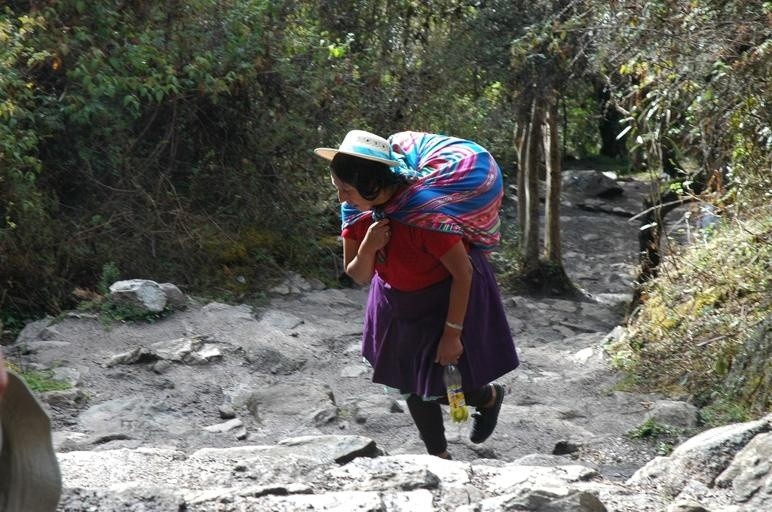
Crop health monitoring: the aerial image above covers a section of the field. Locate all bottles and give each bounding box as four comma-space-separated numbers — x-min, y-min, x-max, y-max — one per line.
441, 363, 468, 424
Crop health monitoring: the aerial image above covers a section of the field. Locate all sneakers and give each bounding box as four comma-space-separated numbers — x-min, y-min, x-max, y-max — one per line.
471, 382, 505, 444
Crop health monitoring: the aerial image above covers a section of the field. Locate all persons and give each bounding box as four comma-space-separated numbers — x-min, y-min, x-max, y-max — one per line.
313, 130, 519, 460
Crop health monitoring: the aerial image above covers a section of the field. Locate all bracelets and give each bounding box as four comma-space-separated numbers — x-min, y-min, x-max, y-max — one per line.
446, 321, 464, 330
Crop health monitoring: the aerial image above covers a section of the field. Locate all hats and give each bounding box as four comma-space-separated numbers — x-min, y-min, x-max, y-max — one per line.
314, 130, 401, 166
